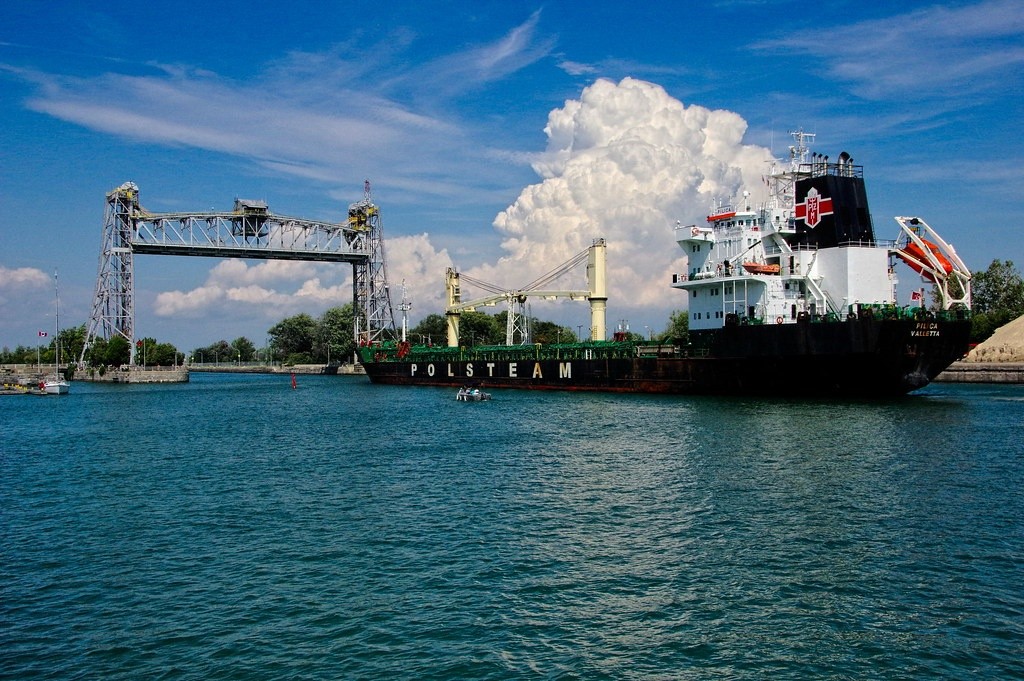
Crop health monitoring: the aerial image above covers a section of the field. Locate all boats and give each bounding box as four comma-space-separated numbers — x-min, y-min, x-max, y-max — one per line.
350, 128, 980, 407
43, 380, 71, 395
455, 385, 492, 403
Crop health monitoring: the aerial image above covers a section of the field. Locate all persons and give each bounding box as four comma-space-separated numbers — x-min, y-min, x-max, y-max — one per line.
40, 381, 44, 390
466, 388, 470, 394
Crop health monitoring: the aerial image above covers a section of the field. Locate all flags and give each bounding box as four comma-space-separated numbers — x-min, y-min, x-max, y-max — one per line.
38, 331, 47, 337
